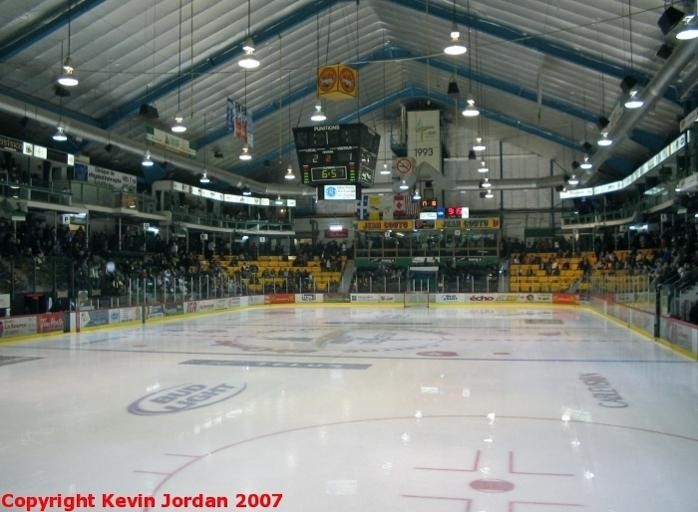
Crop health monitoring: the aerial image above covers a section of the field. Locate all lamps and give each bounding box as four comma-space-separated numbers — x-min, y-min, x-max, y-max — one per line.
51, 1, 698, 207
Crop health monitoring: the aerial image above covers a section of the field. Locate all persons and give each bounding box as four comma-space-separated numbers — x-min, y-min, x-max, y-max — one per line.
0, 162, 698, 317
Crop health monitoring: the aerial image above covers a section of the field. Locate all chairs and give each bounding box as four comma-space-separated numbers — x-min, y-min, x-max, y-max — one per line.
0, 215, 665, 300
